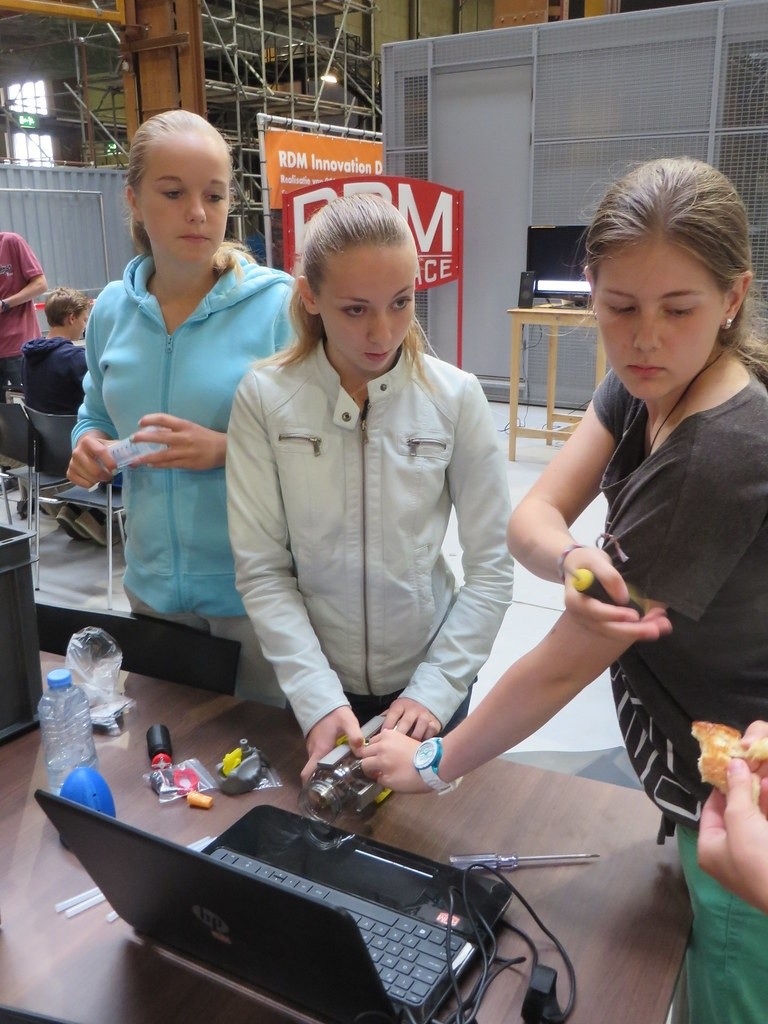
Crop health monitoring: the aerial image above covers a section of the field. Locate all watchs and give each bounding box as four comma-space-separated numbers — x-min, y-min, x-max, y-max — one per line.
414, 737, 464, 794
1, 299, 10, 311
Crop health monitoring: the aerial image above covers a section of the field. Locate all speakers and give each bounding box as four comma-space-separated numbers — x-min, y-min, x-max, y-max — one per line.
519, 271, 534, 308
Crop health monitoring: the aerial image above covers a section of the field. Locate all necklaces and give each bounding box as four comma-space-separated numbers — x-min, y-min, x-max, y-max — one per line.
643, 347, 725, 458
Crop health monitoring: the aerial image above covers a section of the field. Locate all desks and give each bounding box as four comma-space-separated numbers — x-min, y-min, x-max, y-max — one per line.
507, 303, 607, 461
0, 649, 693, 1024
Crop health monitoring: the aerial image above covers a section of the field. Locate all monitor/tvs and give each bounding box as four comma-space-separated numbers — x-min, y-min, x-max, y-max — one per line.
527, 225, 593, 306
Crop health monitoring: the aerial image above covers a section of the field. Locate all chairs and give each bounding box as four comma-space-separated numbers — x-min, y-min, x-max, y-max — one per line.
0, 400, 126, 610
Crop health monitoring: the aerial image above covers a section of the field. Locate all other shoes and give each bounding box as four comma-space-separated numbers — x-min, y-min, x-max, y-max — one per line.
75, 511, 121, 546
55, 505, 91, 540
17, 500, 34, 520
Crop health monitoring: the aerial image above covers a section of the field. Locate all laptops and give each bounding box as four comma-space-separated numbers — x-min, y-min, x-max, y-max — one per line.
35, 785, 514, 1024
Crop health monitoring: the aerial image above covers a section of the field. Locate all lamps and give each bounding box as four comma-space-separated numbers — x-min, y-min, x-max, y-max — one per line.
321, 66, 339, 83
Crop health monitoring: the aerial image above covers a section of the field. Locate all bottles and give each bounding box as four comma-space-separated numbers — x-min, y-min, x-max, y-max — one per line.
37, 668, 100, 797
297, 733, 381, 825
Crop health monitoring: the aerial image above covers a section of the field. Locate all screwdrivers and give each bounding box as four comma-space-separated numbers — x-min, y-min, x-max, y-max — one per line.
449, 852, 600, 869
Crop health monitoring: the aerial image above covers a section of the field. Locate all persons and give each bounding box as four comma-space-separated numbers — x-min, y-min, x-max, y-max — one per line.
226, 194, 514, 787
0, 232, 47, 405
66, 111, 300, 712
21, 287, 89, 415
362, 157, 767, 1024
697, 717, 768, 917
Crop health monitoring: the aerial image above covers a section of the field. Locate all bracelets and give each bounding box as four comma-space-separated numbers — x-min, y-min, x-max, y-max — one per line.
559, 531, 629, 586
